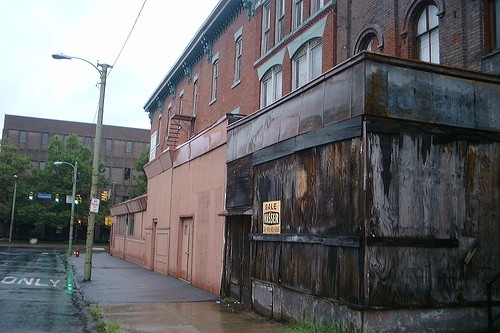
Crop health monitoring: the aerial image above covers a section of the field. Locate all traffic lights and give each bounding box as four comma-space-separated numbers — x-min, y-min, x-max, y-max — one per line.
124, 168, 132, 181
105, 216, 112, 226
29, 192, 33, 200
55, 193, 59, 202
101, 191, 108, 202
75, 194, 82, 204
77, 218, 82, 226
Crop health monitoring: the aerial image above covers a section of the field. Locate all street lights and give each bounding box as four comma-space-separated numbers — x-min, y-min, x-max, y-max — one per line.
53, 160, 78, 256
52, 53, 113, 284
8, 174, 18, 243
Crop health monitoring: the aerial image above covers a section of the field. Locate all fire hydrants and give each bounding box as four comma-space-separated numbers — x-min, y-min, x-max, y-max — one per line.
75, 248, 79, 257
